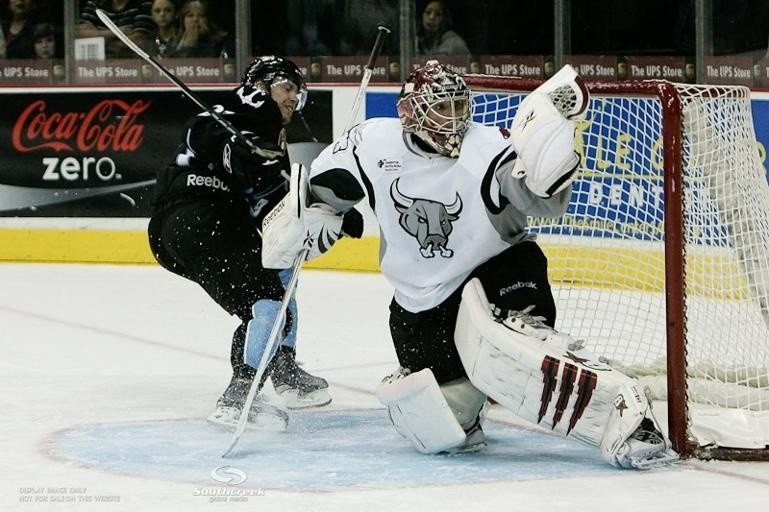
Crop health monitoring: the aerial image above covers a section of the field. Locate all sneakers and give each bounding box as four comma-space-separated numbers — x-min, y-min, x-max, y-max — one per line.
626, 416, 666, 460
438, 423, 488, 455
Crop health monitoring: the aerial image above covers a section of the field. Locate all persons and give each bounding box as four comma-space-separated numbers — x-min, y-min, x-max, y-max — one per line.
416, 0, 476, 58
261, 63, 666, 471
1, 0, 233, 60
146, 56, 328, 422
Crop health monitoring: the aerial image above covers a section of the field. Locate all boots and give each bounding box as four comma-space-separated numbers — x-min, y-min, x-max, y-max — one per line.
216, 345, 328, 425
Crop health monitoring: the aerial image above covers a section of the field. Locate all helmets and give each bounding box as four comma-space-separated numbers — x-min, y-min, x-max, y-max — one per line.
240, 55, 308, 112
396, 59, 473, 158
